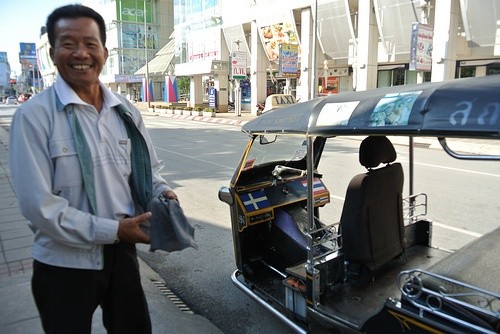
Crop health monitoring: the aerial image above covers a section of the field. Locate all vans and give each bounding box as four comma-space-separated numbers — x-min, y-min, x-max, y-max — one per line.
17, 94, 32, 102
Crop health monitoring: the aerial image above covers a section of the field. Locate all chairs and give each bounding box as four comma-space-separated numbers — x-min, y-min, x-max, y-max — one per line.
338, 136, 406, 285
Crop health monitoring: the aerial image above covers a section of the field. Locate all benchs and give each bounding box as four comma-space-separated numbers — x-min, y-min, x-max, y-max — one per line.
397, 227, 500, 334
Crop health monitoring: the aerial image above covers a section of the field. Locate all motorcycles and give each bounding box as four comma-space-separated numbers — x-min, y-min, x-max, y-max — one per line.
216, 73, 499, 333
255, 101, 266, 115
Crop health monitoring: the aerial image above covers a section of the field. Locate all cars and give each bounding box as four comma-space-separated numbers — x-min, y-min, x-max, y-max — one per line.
5, 96, 17, 105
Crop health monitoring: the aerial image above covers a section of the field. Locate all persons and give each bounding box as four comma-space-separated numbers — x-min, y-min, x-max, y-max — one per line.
9, 4, 179, 334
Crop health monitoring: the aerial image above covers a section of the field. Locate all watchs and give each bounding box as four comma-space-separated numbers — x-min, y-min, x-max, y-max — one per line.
114, 236, 119, 243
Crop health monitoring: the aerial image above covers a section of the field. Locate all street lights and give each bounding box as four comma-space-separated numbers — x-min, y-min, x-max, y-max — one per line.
111, 19, 153, 107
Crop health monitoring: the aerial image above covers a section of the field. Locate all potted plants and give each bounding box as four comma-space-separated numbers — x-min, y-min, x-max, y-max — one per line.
148, 105, 216, 117
